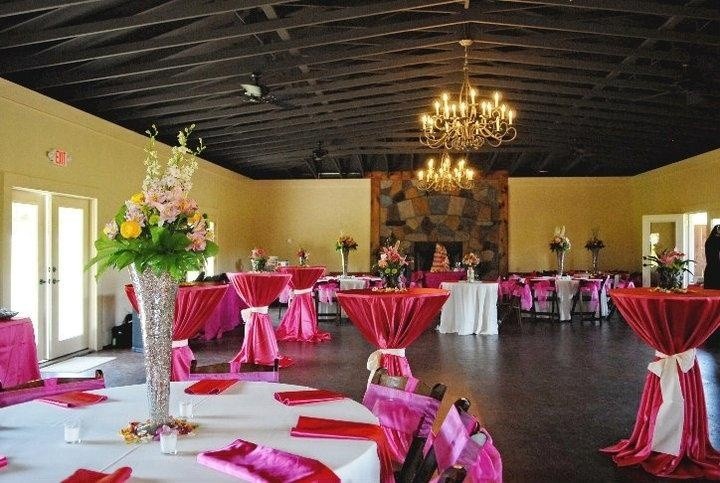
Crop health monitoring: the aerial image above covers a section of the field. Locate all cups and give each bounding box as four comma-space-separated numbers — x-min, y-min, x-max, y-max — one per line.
184, 269, 201, 283
64, 422, 82, 445
178, 400, 200, 420
159, 430, 179, 455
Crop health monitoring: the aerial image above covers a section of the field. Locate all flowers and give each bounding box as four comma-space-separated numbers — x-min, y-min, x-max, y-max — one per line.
462, 252, 480, 268
549, 226, 571, 253
336, 236, 358, 251
377, 240, 409, 277
84, 125, 219, 280
643, 248, 697, 275
296, 248, 310, 262
248, 247, 270, 262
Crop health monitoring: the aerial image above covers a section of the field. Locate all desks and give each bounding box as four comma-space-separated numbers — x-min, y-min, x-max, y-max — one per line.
609, 288, 720, 457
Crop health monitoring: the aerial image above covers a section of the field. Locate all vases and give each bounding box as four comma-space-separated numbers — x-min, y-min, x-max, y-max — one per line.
340, 249, 348, 276
252, 261, 265, 272
557, 251, 564, 279
591, 249, 599, 275
467, 267, 474, 282
655, 267, 683, 289
130, 260, 179, 435
585, 233, 605, 251
299, 257, 305, 266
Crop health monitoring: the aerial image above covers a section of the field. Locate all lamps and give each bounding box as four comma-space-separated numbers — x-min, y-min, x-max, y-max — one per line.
419, 23, 517, 150
313, 149, 327, 161
240, 84, 269, 98
416, 150, 475, 192
